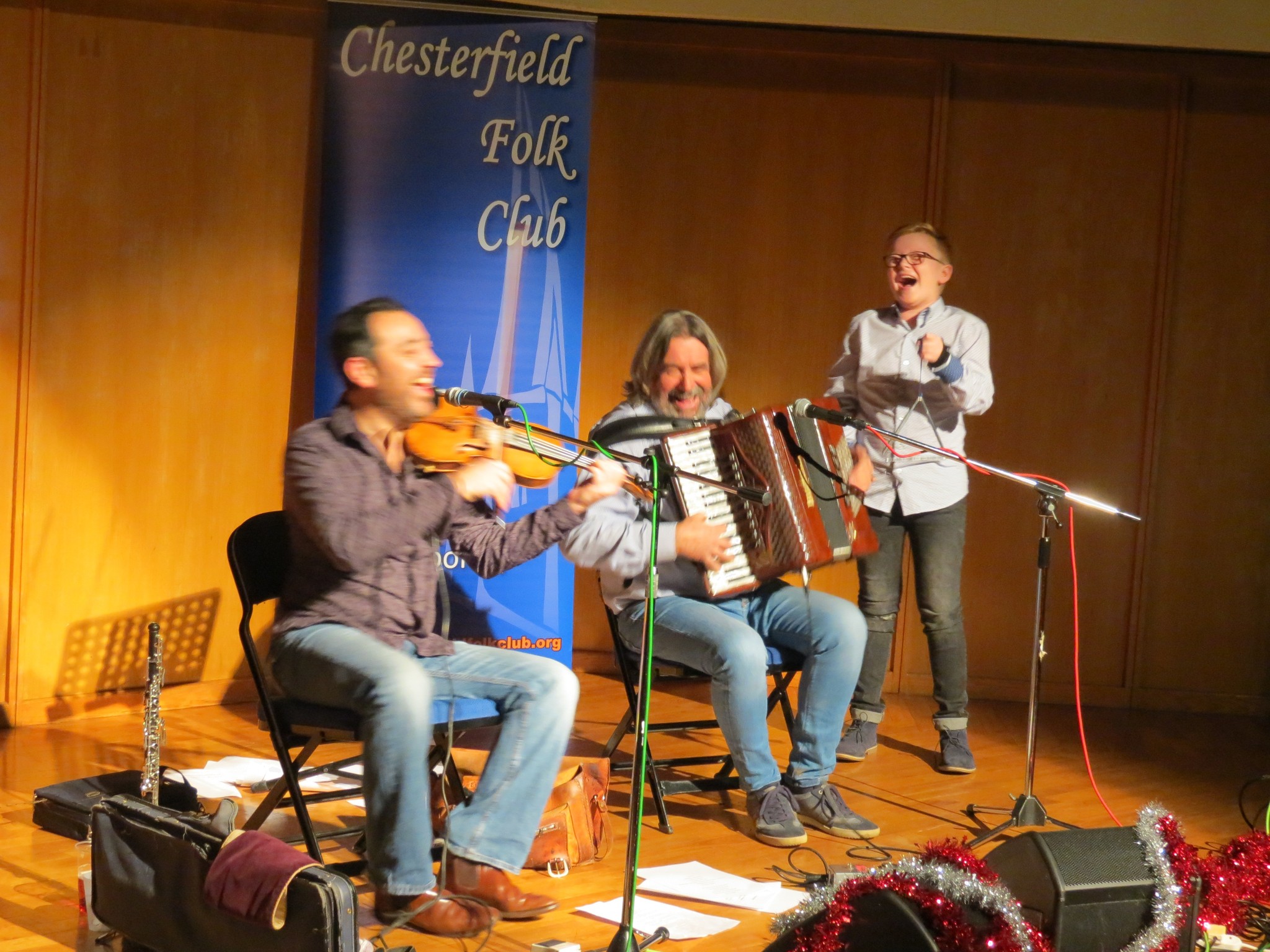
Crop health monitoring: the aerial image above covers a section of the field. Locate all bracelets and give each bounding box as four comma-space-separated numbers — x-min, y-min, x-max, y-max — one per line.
928, 353, 964, 384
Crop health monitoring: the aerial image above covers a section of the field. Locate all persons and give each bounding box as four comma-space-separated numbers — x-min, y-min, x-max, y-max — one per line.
823, 222, 995, 774
559, 309, 882, 849
269, 298, 627, 936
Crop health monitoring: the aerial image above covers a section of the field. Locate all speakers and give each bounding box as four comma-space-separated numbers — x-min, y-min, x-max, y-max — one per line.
763, 828, 1203, 951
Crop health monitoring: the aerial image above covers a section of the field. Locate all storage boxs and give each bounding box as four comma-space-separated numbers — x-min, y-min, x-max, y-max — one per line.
32, 767, 198, 843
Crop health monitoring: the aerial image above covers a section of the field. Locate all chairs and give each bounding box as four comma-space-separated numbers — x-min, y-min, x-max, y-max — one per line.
599, 572, 807, 835
222, 510, 500, 878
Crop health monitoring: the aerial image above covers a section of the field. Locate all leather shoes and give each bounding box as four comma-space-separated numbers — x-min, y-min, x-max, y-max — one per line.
376, 884, 501, 937
437, 851, 559, 919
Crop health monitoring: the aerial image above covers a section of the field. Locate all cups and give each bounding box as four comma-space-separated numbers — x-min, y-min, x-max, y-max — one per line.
82, 870, 110, 932
75, 841, 92, 914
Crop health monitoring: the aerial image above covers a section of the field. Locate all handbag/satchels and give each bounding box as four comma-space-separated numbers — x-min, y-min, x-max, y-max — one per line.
432, 748, 611, 877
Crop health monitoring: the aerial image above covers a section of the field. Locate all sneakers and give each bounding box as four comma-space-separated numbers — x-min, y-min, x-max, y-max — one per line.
746, 781, 808, 847
932, 725, 977, 773
787, 782, 880, 839
835, 712, 877, 761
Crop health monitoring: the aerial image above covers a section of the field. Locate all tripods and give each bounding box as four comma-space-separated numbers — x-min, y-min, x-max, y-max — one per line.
862, 425, 1142, 852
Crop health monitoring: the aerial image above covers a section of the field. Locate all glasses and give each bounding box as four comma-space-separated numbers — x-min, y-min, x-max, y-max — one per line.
883, 251, 945, 266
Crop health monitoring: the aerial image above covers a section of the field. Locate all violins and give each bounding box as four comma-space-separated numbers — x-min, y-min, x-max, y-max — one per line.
403, 396, 659, 506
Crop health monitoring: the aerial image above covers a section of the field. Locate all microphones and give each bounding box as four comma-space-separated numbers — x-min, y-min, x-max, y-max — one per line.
444, 387, 519, 408
793, 398, 867, 430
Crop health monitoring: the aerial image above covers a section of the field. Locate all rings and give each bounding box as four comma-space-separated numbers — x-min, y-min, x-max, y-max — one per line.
713, 555, 718, 561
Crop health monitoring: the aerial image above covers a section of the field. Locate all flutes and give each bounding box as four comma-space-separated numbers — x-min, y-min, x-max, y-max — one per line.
137, 622, 167, 806
